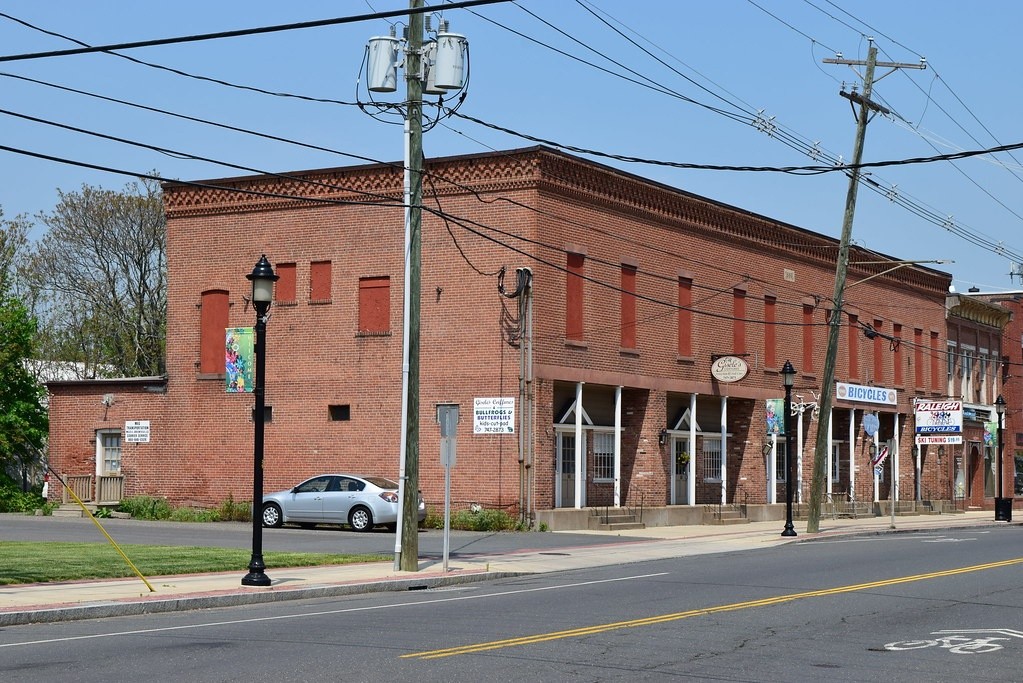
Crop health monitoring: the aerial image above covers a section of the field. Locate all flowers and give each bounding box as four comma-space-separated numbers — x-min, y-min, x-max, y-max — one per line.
676, 450, 690, 468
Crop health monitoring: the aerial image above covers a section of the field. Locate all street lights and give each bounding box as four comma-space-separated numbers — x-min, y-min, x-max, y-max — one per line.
994, 394, 1007, 521
242, 255, 281, 587
778, 359, 798, 537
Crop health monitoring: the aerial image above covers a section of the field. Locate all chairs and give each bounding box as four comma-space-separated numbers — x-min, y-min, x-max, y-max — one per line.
330, 481, 357, 491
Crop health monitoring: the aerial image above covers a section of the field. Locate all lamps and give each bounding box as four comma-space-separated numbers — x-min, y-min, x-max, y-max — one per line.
869, 441, 878, 455
761, 440, 775, 455
912, 444, 919, 458
938, 444, 945, 457
658, 428, 670, 446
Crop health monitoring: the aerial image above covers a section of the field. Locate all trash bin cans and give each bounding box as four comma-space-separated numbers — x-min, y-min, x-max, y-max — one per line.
994, 497, 1013, 522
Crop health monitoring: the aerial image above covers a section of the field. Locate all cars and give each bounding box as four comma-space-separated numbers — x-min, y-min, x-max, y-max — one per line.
250, 474, 427, 533
